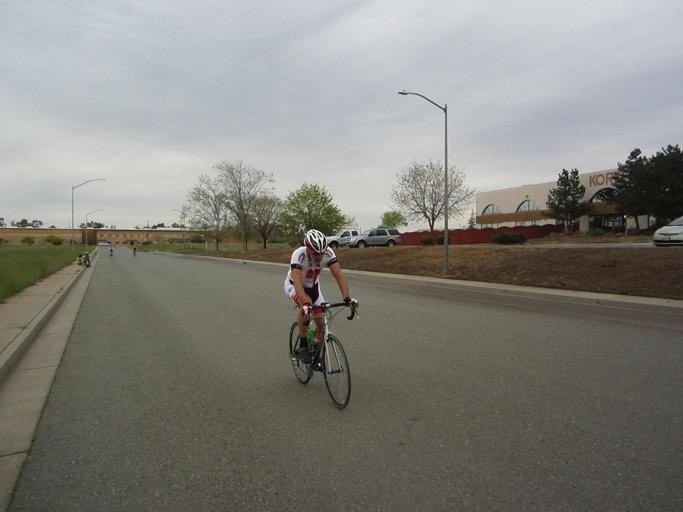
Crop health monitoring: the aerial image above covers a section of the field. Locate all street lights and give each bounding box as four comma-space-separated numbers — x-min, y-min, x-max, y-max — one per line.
397, 89, 450, 278
173, 208, 186, 246
72, 178, 107, 247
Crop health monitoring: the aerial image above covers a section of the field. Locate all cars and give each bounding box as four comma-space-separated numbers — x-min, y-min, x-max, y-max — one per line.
653, 216, 683, 247
325, 229, 400, 250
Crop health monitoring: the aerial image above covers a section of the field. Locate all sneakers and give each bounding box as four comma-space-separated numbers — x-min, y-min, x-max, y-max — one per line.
295, 344, 311, 364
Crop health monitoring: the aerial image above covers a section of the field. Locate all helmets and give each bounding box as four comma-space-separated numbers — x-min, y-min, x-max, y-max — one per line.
304, 229, 327, 254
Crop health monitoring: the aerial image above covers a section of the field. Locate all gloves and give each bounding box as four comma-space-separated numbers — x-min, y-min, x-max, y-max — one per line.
302, 302, 312, 317
344, 297, 357, 304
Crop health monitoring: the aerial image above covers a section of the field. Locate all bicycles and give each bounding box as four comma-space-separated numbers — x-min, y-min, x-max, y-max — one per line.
289, 302, 359, 409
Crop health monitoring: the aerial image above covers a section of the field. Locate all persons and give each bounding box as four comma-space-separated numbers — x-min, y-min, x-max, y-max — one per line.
284, 229, 358, 372
